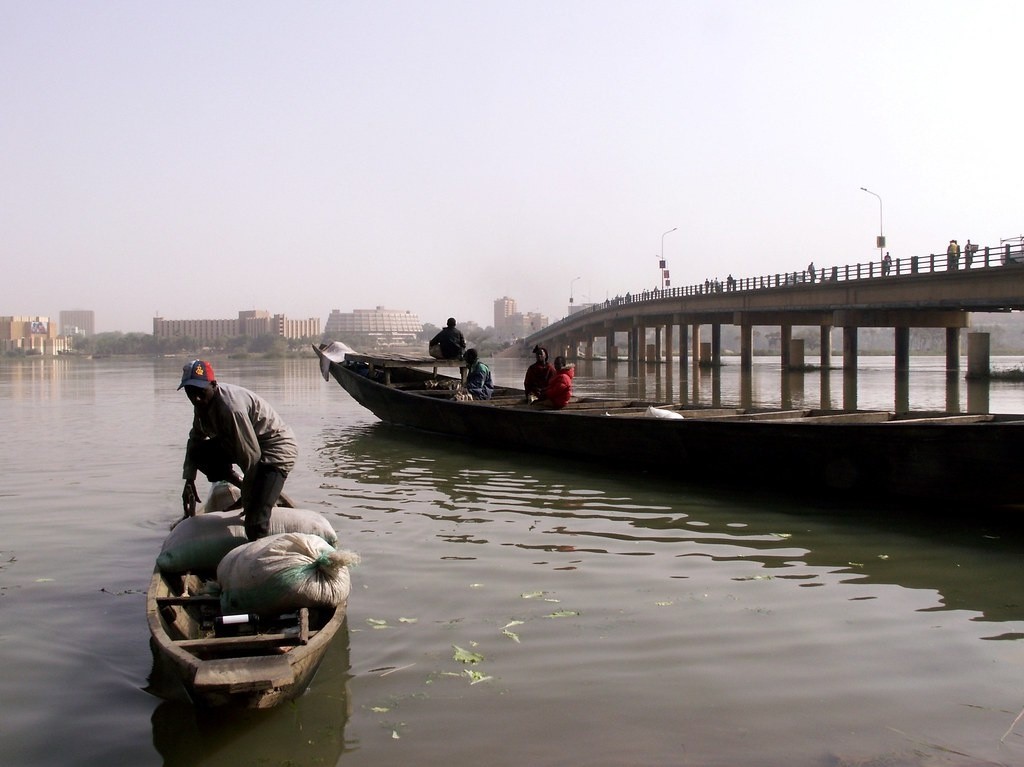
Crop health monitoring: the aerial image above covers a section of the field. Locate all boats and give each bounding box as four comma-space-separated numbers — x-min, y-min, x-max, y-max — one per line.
309, 327, 1023, 519
144, 488, 353, 710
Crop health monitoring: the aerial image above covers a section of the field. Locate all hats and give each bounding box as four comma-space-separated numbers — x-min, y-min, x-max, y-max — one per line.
177, 358, 215, 391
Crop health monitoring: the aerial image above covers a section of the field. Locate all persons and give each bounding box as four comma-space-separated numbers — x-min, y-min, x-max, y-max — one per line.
965, 239, 973, 269
449, 348, 494, 401
524, 344, 556, 406
949, 240, 958, 271
176, 359, 299, 543
430, 317, 467, 360
529, 356, 575, 411
808, 262, 816, 284
884, 252, 892, 276
953, 240, 961, 270
594, 274, 737, 312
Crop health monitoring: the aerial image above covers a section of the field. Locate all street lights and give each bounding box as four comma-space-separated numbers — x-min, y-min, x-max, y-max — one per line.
660, 227, 678, 289
861, 187, 884, 262
